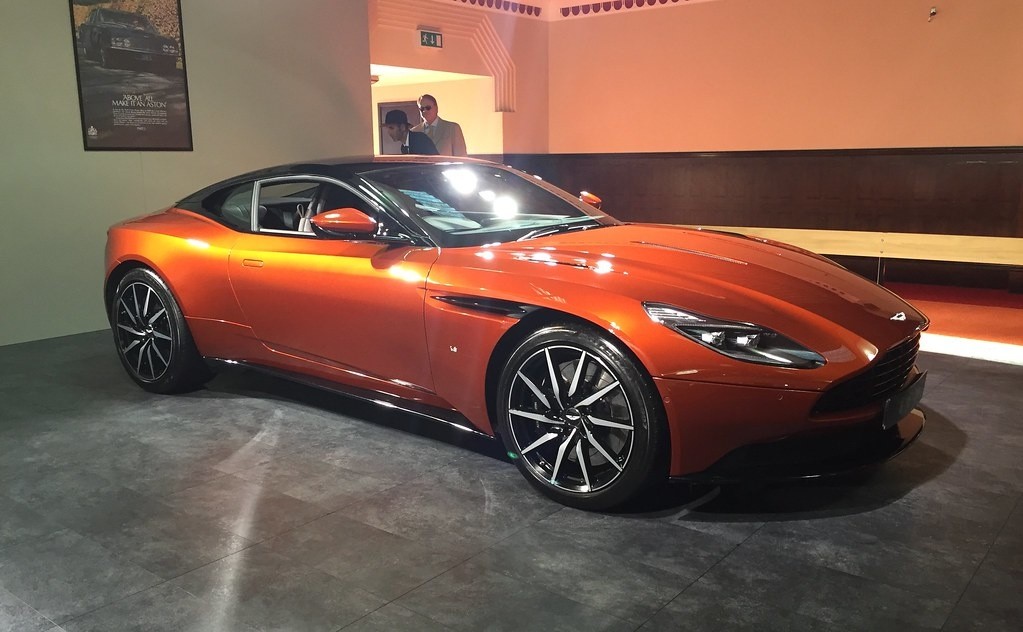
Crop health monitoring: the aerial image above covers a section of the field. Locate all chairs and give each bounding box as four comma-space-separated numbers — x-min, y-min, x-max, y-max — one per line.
297, 182, 359, 232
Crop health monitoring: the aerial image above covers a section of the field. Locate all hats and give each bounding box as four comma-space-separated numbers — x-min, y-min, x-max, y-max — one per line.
380, 110, 414, 128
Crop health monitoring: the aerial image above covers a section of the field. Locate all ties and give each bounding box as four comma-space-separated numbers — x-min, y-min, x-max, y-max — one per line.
404, 146, 411, 154
428, 125, 433, 138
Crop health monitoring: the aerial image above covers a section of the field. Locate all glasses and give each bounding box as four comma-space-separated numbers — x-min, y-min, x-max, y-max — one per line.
418, 103, 436, 112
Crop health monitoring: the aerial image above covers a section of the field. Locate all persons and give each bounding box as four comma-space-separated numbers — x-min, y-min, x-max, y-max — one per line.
379, 110, 440, 155
409, 95, 469, 156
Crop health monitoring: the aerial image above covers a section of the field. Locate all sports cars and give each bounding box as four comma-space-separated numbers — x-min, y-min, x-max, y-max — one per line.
101, 153, 929, 515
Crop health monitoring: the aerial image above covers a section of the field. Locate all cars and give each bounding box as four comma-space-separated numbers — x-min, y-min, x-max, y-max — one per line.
78, 9, 180, 73
